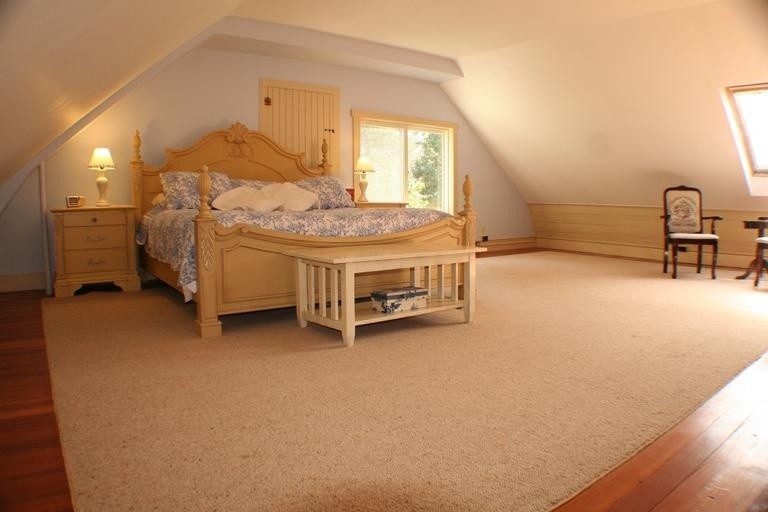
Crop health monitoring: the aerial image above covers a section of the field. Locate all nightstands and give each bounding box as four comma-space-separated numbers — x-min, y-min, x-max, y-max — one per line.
51, 205, 142, 296
355, 203, 409, 208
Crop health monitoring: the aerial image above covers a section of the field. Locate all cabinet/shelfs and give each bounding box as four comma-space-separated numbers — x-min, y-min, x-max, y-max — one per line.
283, 245, 488, 347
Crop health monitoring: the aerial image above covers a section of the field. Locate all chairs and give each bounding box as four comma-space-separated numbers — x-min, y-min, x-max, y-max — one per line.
660, 185, 724, 279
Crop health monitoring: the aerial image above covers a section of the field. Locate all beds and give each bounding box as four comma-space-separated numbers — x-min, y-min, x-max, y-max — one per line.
129, 121, 481, 338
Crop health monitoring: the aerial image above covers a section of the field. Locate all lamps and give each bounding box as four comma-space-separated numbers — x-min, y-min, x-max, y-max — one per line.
88, 148, 116, 206
354, 156, 376, 202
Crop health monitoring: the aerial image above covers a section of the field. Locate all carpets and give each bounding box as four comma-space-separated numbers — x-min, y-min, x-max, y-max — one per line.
40, 251, 768, 511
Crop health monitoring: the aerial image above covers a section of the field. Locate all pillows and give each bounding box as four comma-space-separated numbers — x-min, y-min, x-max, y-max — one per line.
159, 171, 356, 209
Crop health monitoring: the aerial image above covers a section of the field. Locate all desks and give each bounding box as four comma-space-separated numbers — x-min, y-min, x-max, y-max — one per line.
736, 221, 768, 286
